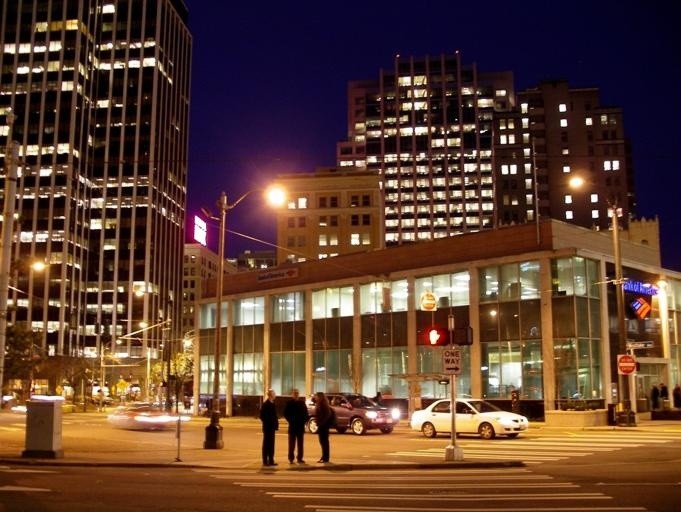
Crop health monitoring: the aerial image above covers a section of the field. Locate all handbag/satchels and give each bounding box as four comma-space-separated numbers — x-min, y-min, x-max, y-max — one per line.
327, 407, 337, 430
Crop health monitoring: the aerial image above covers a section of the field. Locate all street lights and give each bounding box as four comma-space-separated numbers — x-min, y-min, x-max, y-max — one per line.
203, 185, 288, 449
566, 175, 636, 427
102, 339, 123, 386
139, 320, 164, 413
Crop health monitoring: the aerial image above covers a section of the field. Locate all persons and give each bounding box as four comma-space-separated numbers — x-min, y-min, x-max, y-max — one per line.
313, 391, 333, 463
660, 383, 669, 399
651, 384, 659, 409
373, 392, 384, 406
259, 389, 278, 465
282, 388, 310, 464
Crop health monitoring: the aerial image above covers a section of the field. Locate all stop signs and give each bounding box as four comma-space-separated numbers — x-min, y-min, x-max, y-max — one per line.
421, 292, 437, 312
618, 355, 636, 374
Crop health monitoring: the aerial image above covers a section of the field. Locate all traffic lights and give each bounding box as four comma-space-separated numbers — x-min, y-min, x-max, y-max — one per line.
427, 328, 449, 346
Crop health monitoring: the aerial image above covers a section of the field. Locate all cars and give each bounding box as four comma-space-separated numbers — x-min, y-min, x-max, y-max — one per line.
199, 394, 261, 418
410, 397, 528, 438
423, 385, 618, 400
92, 394, 195, 432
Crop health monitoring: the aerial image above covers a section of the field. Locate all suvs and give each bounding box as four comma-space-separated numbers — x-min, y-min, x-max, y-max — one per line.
305, 392, 400, 436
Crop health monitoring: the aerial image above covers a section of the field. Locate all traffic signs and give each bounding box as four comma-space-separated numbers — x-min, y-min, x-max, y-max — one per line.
442, 350, 461, 375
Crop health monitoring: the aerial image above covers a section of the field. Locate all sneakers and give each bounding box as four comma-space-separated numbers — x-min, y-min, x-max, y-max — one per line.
263, 457, 329, 466
